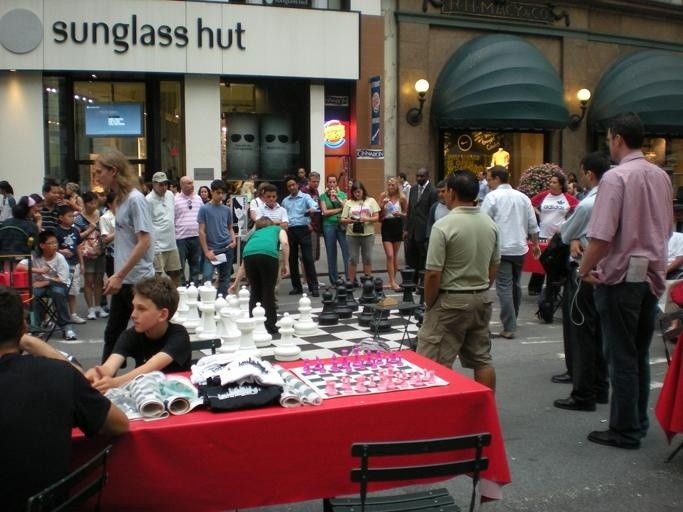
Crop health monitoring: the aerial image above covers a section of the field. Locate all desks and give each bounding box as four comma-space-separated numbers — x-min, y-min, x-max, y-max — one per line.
361, 296, 423, 351
69, 351, 511, 511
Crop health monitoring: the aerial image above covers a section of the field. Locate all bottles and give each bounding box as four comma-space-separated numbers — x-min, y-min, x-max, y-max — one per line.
386, 199, 399, 217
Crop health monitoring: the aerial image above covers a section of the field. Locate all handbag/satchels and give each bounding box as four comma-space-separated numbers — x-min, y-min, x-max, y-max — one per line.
539, 232, 579, 287
379, 192, 386, 219
353, 222, 364, 233
248, 199, 258, 229
338, 223, 346, 232
73, 213, 103, 260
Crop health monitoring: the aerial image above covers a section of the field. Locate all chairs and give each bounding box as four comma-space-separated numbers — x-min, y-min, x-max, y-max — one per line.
0, 225, 34, 315
323, 432, 492, 512
35, 264, 76, 342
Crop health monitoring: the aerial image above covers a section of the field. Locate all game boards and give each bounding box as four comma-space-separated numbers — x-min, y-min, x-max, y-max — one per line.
289, 358, 450, 400
201, 298, 419, 361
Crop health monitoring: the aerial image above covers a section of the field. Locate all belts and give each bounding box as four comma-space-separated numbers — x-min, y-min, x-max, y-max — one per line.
438, 286, 490, 294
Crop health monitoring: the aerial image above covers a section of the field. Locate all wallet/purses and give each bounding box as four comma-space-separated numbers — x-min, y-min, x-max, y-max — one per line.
625, 255, 649, 283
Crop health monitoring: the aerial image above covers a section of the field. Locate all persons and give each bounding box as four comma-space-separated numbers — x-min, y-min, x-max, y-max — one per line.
575, 110, 674, 448
1, 179, 119, 339
96, 150, 155, 374
379, 166, 613, 411
667, 229, 683, 280
656, 317, 680, 345
0, 284, 129, 512
486, 148, 510, 169
135, 168, 381, 333
91, 273, 192, 395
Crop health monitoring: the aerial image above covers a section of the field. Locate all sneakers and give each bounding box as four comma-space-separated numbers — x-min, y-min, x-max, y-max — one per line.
69, 315, 86, 324
302, 281, 309, 290
95, 308, 110, 318
319, 282, 325, 287
664, 327, 680, 335
289, 289, 302, 295
62, 329, 77, 340
395, 287, 416, 292
87, 308, 96, 320
500, 330, 513, 339
311, 289, 319, 297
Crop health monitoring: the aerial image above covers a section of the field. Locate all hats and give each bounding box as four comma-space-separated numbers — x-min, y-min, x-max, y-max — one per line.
152, 172, 169, 183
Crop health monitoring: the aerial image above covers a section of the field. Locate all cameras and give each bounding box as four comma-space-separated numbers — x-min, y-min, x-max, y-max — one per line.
351, 216, 361, 221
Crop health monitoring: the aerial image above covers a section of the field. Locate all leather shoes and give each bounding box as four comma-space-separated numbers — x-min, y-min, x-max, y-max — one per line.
553, 396, 595, 410
551, 372, 573, 383
587, 429, 640, 449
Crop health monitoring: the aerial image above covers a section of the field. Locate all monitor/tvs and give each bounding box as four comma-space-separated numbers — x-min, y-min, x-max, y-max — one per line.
83, 102, 145, 137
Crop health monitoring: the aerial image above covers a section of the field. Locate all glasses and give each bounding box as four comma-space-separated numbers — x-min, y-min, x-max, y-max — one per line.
187, 200, 192, 209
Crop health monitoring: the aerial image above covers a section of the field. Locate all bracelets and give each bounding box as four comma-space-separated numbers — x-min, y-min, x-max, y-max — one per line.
575, 265, 590, 279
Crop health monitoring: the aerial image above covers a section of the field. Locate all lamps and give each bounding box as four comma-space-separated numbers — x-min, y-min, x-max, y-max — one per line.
407, 79, 430, 127
569, 86, 592, 132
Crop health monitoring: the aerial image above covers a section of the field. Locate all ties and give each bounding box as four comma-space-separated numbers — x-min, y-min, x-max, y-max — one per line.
418, 186, 423, 200
